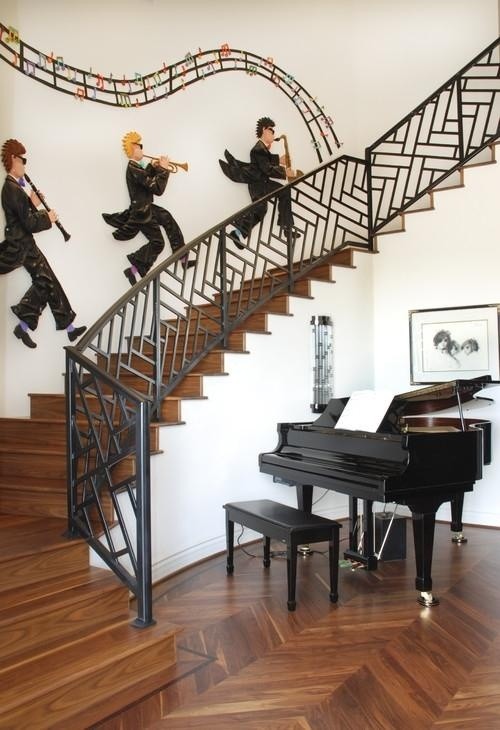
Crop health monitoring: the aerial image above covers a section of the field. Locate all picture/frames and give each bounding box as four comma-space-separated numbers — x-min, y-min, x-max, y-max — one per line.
408, 303, 500, 386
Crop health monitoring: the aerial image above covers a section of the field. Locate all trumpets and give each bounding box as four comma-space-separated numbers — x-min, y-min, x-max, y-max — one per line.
143, 154, 188, 173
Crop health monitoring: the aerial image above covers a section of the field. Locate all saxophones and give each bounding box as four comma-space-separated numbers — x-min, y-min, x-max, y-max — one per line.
274, 135, 304, 185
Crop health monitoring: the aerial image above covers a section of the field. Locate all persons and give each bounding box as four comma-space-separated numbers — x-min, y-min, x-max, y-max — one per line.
219, 117, 301, 251
461, 338, 480, 368
1, 140, 87, 348
446, 339, 459, 357
429, 330, 459, 370
100, 131, 196, 289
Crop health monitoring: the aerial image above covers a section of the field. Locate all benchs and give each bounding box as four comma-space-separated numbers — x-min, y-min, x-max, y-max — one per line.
223, 500, 342, 611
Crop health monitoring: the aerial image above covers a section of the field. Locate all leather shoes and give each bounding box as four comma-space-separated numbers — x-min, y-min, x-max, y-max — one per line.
229, 229, 246, 250
123, 267, 137, 286
65, 325, 88, 342
181, 259, 199, 270
283, 229, 303, 238
12, 323, 37, 349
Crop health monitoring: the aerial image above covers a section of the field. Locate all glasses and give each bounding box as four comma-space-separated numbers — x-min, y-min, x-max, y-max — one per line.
130, 139, 145, 150
12, 151, 27, 166
267, 126, 275, 135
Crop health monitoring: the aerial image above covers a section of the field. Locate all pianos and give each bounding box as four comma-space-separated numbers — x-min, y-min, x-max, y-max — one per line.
259, 375, 500, 606
